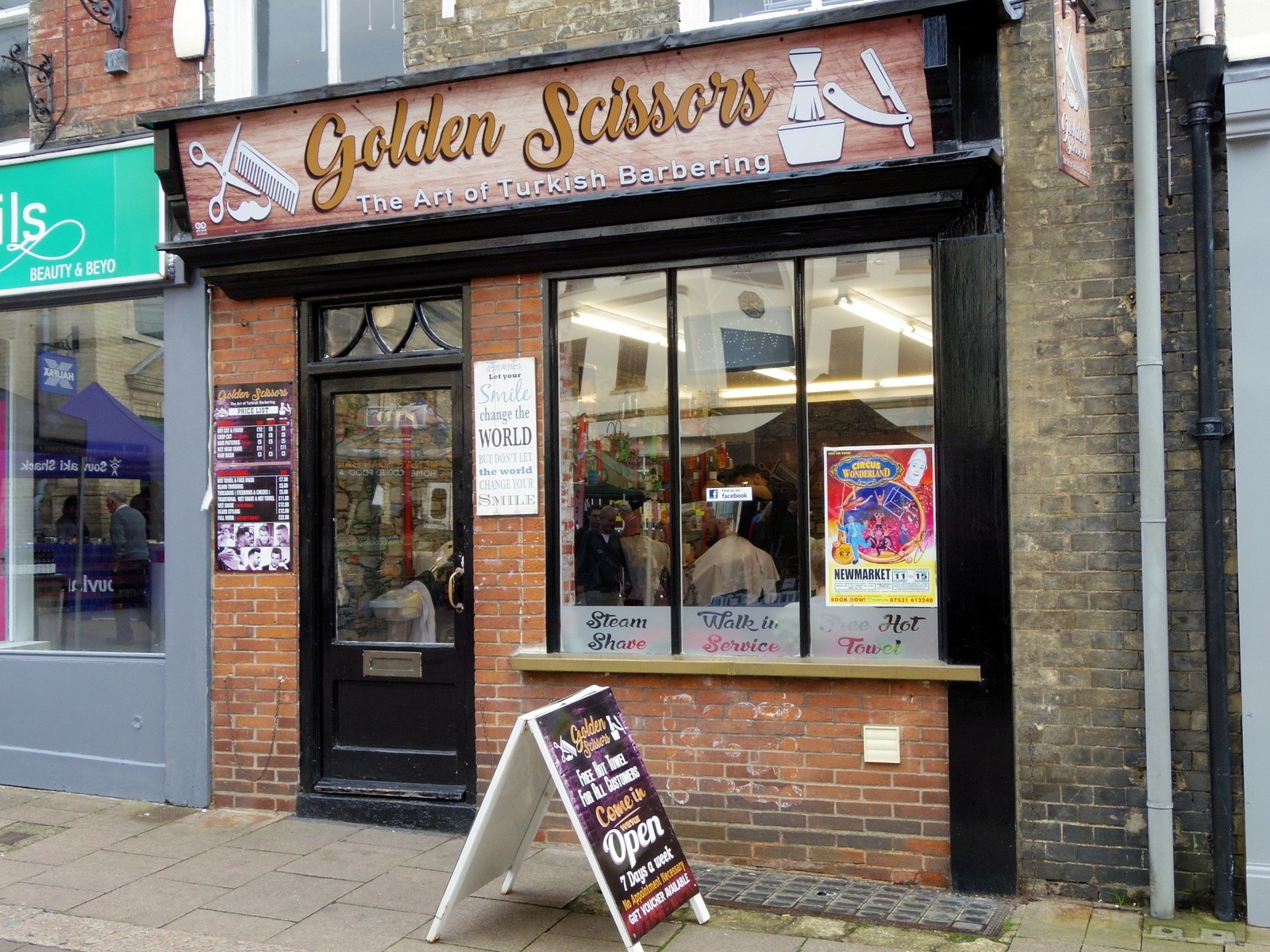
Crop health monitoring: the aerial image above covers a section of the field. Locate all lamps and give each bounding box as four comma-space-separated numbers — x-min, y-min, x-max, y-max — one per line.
172, 0, 210, 62
571, 302, 797, 382
834, 287, 934, 347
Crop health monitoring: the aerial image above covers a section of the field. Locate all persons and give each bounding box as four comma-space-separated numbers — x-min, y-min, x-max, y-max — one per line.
218, 524, 291, 571
575, 464, 798, 607
53, 491, 164, 645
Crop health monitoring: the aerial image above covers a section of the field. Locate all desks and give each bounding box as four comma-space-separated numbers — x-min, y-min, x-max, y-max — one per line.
31, 544, 142, 622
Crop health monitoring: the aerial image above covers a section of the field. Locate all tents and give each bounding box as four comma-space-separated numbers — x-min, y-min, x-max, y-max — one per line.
573, 441, 656, 504
1, 382, 164, 650
696, 370, 932, 534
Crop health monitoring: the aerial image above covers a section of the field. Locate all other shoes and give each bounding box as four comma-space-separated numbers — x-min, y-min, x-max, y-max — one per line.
107, 637, 133, 645
154, 635, 160, 644
